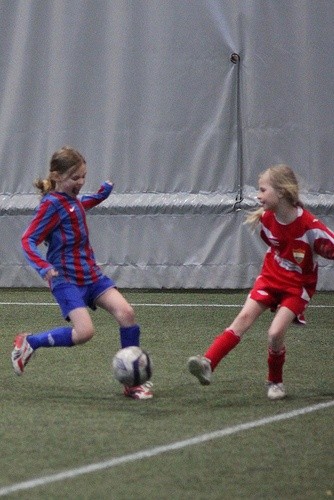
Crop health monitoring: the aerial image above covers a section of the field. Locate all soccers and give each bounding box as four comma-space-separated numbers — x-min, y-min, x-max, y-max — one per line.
112, 346, 154, 388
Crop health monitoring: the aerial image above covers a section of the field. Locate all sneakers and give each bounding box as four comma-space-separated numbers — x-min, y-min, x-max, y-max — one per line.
188, 353, 213, 387
10, 332, 35, 375
267, 383, 285, 400
124, 383, 154, 402
